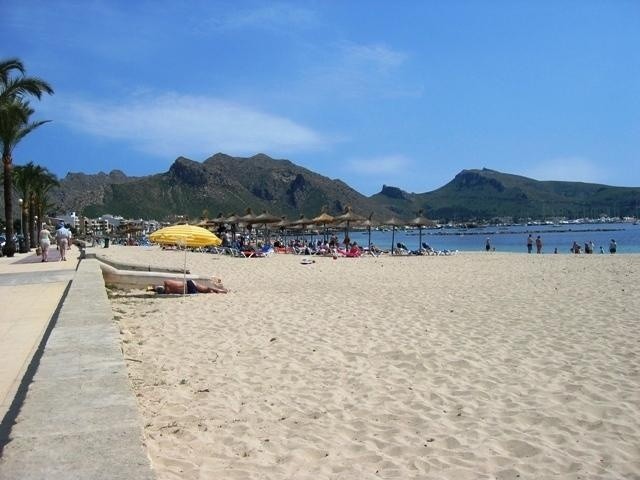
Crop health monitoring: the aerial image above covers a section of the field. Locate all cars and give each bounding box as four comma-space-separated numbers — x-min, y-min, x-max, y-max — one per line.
0, 232, 31, 256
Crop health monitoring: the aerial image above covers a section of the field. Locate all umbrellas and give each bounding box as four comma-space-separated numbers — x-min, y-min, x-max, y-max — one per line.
147, 222, 223, 297
194, 208, 437, 256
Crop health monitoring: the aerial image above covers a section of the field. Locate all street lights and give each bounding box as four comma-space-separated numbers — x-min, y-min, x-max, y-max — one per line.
17, 198, 24, 234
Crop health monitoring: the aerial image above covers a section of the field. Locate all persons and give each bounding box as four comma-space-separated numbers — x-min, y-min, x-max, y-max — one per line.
485, 237, 491, 251
38, 224, 55, 263
153, 279, 229, 295
569, 238, 617, 255
535, 235, 542, 254
54, 221, 71, 262
526, 234, 535, 254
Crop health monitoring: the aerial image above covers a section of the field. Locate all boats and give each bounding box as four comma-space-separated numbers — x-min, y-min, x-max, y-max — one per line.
375, 206, 640, 235
